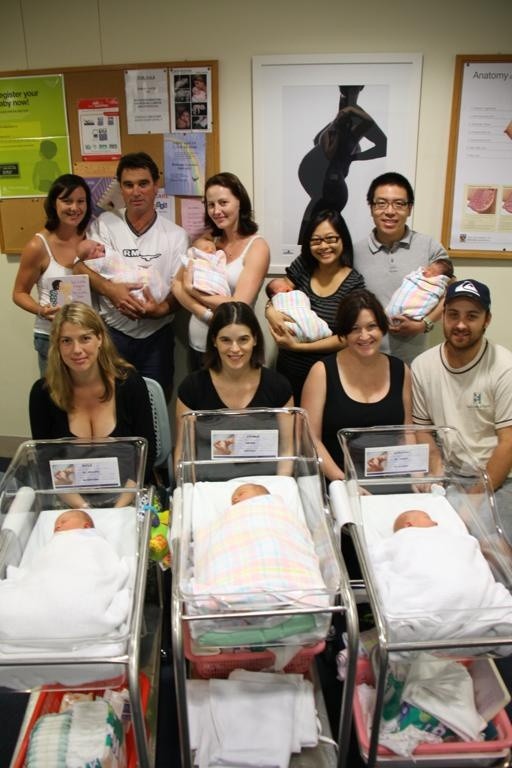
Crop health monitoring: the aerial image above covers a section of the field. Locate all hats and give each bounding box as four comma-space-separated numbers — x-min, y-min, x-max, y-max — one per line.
442, 279, 492, 314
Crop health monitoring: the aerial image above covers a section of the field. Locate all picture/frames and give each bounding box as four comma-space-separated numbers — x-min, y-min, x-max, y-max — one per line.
436, 51, 511, 261
250, 51, 424, 276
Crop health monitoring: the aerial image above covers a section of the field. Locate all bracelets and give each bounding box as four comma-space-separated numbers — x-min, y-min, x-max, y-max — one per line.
423, 316, 434, 334
38, 306, 46, 321
202, 308, 212, 322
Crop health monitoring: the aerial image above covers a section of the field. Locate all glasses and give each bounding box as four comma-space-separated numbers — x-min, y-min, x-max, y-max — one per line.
308, 235, 340, 247
370, 198, 412, 211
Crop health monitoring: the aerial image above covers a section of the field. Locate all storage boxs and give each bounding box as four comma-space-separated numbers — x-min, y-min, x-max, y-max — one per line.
357, 724, 510, 767
184, 618, 327, 680
12, 672, 149, 768
344, 651, 512, 752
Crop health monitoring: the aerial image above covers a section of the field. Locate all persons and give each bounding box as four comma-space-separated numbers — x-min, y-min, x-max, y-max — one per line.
264, 208, 365, 408
231, 483, 271, 505
352, 170, 456, 370
50, 279, 64, 307
299, 286, 425, 496
366, 451, 388, 473
72, 150, 190, 406
174, 74, 208, 129
393, 510, 438, 533
75, 239, 170, 305
12, 173, 101, 378
54, 464, 75, 486
265, 278, 334, 343
384, 258, 454, 327
409, 278, 512, 596
53, 509, 95, 532
173, 299, 296, 483
213, 433, 235, 456
171, 171, 271, 375
178, 236, 232, 297
28, 300, 157, 509
